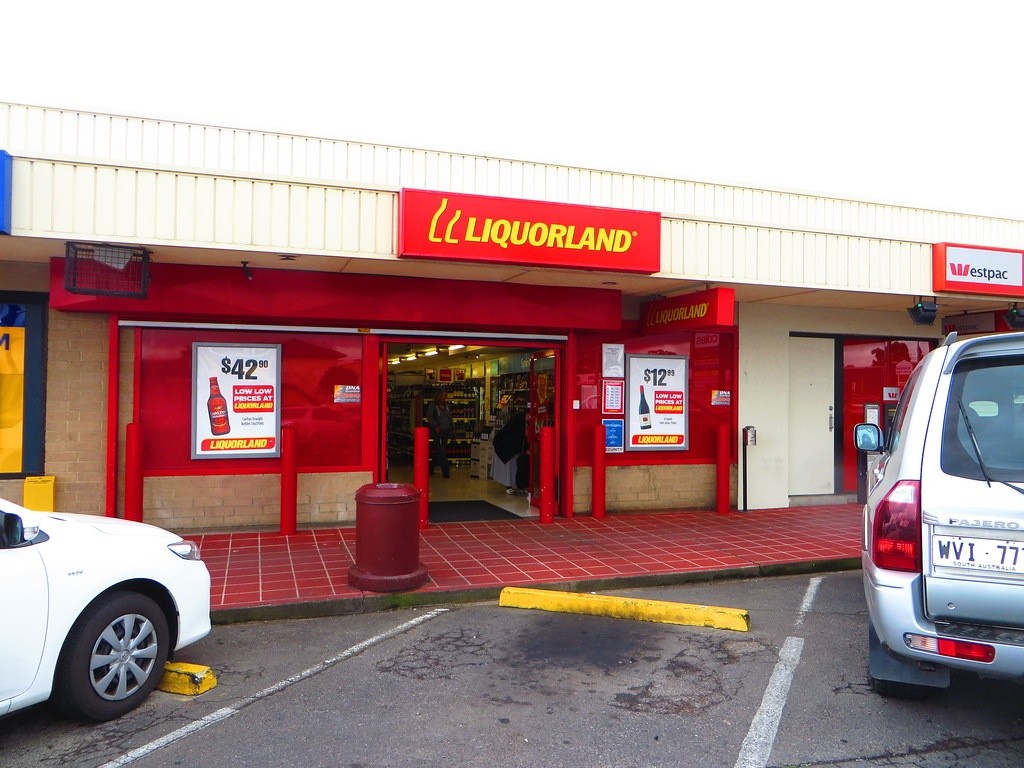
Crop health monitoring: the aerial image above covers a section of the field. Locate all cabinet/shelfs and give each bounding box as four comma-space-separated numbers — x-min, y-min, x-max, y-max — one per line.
387, 369, 555, 473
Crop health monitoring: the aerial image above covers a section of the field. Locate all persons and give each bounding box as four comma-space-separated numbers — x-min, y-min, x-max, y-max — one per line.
430, 390, 452, 478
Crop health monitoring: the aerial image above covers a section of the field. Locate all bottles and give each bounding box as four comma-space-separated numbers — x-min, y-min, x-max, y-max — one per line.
639, 385, 651, 430
388, 372, 554, 459
207, 377, 229, 435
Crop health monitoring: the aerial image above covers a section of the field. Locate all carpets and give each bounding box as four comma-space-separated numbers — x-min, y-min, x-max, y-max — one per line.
428, 500, 524, 523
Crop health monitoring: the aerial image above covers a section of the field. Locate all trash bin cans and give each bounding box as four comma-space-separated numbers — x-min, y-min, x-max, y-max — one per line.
348, 483, 428, 591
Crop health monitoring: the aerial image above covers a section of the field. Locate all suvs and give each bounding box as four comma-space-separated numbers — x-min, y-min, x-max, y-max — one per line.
853, 330, 1024, 700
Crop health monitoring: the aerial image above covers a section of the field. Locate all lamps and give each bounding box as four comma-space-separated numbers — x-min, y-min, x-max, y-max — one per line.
906, 295, 938, 326
1003, 302, 1024, 330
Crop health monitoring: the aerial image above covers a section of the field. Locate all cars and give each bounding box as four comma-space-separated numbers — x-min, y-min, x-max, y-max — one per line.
0, 496, 213, 723
